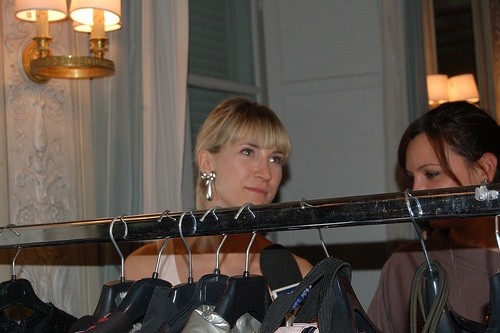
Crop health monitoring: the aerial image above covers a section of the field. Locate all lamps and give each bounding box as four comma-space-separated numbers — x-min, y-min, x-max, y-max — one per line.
15, 2, 121, 81
428, 75, 478, 113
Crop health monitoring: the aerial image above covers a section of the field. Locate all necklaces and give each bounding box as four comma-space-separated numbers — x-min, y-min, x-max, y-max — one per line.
449, 228, 500, 325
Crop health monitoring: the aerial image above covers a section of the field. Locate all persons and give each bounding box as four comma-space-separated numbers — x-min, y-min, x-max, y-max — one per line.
366, 101, 500, 333
125, 98, 315, 327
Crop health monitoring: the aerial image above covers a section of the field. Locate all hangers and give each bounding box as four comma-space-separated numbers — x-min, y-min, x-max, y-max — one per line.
0, 180, 500, 333
